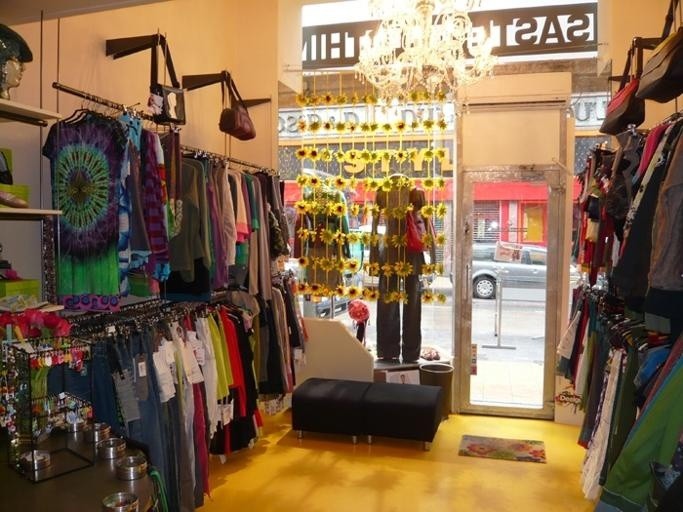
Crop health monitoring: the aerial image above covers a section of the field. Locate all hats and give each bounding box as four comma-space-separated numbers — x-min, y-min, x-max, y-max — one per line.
1, 24, 33, 64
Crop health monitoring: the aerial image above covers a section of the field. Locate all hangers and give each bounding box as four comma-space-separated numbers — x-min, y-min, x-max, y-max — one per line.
612, 320, 673, 351
65, 91, 167, 135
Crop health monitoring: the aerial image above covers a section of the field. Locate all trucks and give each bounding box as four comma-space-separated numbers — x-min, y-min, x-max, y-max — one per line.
360, 222, 436, 290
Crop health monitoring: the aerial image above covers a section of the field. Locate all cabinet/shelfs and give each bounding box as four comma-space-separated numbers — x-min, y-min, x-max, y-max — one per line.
0, 98, 64, 314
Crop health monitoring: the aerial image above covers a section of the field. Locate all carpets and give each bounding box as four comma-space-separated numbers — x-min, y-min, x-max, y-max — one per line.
459, 435, 547, 463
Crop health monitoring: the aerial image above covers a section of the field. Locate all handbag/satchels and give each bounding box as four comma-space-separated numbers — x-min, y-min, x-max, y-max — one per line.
219, 107, 256, 142
599, 80, 646, 136
405, 210, 436, 251
635, 26, 683, 104
149, 83, 187, 126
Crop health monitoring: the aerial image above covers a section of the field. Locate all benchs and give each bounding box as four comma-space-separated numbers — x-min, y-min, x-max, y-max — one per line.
292, 378, 444, 451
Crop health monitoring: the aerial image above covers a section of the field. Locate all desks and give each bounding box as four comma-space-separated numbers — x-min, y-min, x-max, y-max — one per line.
0, 432, 158, 512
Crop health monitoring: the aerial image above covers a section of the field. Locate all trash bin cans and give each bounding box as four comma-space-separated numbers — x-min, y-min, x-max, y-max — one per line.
420, 364, 455, 417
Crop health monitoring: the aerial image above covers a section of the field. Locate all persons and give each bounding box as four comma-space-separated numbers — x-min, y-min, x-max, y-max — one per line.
370, 171, 438, 363
293, 182, 353, 296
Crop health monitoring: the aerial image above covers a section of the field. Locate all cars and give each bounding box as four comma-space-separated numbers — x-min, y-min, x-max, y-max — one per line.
450, 239, 602, 300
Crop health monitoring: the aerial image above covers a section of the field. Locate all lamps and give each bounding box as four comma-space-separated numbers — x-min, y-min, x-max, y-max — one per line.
354, 0, 498, 116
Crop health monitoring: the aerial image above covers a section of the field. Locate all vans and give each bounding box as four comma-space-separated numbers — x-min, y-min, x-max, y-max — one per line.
284, 207, 364, 318
